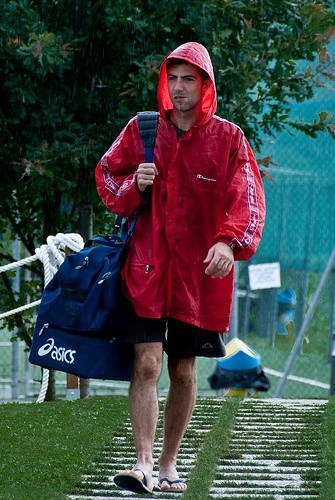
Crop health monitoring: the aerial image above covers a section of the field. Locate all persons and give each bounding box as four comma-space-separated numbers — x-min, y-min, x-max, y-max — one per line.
95, 42, 265, 496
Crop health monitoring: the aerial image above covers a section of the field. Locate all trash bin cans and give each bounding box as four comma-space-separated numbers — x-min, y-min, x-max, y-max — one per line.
275, 289, 297, 338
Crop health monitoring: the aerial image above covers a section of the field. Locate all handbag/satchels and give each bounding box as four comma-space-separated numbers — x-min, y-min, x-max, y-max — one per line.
27, 229, 133, 381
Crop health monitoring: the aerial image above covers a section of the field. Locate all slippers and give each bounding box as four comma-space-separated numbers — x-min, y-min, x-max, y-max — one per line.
114, 470, 152, 496
153, 477, 188, 492
